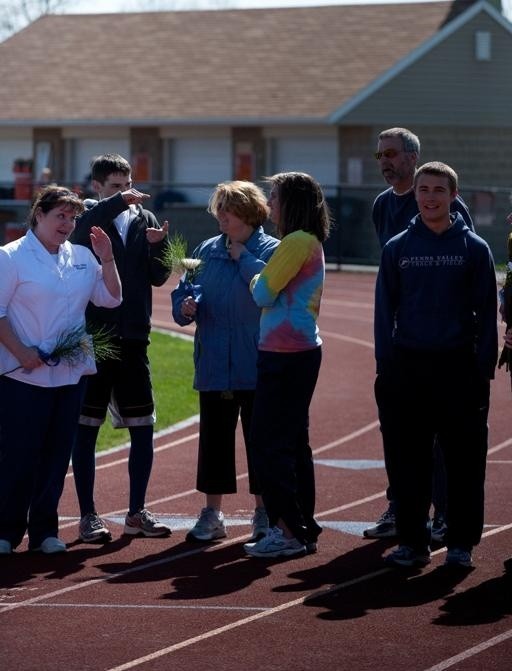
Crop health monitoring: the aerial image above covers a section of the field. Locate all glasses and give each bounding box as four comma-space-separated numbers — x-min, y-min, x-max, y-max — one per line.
374, 147, 411, 162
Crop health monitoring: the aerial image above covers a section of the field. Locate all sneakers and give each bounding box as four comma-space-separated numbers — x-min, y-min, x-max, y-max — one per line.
244, 525, 318, 560
250, 505, 269, 539
184, 506, 227, 544
442, 544, 476, 572
364, 509, 396, 539
429, 518, 447, 542
1, 539, 12, 554
122, 508, 173, 538
387, 545, 432, 566
77, 513, 113, 542
27, 535, 67, 554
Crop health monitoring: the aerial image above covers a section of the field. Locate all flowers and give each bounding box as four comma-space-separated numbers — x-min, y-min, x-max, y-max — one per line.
152, 229, 202, 299
0, 318, 124, 379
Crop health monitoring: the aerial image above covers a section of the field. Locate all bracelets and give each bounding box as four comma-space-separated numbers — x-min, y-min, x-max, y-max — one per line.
101, 258, 114, 264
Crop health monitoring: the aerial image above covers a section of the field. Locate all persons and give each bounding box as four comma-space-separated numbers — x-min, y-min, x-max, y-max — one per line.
1, 186, 122, 553
243, 171, 331, 559
362, 128, 474, 542
498, 212, 512, 374
71, 154, 172, 543
171, 180, 283, 547
374, 159, 498, 568
